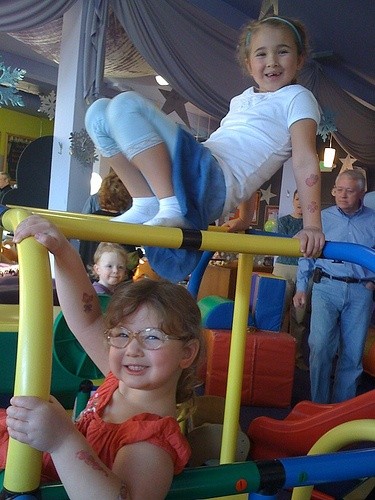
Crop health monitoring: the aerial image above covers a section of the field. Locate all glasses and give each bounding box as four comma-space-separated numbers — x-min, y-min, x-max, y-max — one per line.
104, 326, 191, 351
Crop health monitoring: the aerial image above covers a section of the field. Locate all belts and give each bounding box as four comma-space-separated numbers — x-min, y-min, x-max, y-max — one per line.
313, 267, 373, 284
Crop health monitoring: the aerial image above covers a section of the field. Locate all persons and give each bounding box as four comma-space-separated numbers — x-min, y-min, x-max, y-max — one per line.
83, 14, 327, 260
268, 189, 315, 369
82, 172, 101, 215
293, 170, 374, 403
0, 172, 13, 207
5, 216, 205, 500
78, 172, 137, 270
91, 241, 129, 295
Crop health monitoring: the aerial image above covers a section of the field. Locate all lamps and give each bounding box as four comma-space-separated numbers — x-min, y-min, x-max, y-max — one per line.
323, 134, 336, 168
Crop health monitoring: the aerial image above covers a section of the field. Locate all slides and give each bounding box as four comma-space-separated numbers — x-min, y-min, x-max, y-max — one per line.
247, 389, 375, 455
